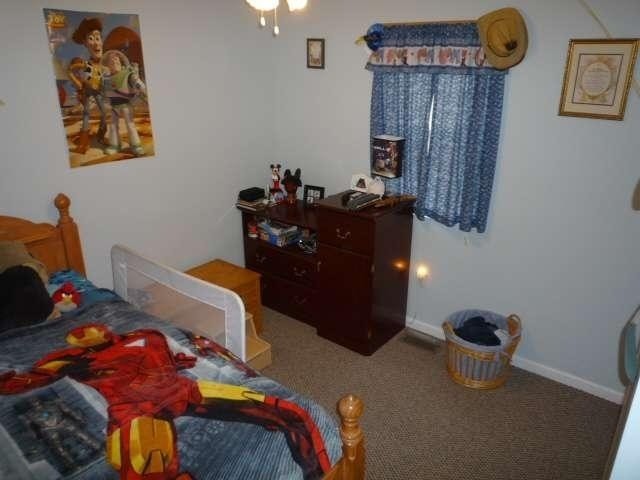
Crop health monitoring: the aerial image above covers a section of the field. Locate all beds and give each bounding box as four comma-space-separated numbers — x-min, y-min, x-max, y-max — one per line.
1, 192, 369, 480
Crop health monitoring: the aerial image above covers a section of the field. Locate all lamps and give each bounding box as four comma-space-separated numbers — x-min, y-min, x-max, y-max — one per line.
248, 0, 310, 34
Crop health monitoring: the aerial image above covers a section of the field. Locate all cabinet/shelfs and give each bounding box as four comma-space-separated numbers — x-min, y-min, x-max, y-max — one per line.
236, 191, 419, 359
133, 253, 264, 337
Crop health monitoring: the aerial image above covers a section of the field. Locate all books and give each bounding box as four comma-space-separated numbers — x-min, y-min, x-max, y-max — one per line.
235, 185, 300, 247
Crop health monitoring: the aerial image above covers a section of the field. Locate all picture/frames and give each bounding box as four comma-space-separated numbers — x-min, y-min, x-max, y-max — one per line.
305, 37, 325, 70
555, 38, 639, 121
